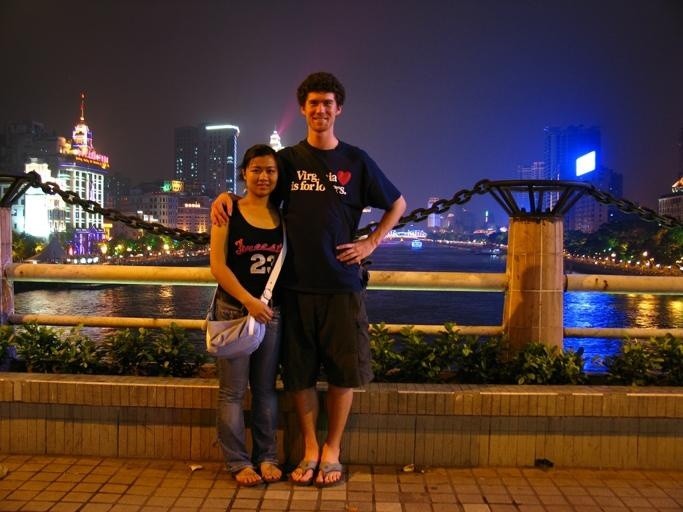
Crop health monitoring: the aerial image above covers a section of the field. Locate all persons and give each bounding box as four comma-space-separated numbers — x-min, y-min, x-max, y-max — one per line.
206, 72, 409, 488
208, 143, 288, 488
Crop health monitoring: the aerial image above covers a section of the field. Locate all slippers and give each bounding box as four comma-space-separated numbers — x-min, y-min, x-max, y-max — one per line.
261, 462, 283, 483
233, 468, 262, 487
289, 458, 316, 485
313, 463, 343, 487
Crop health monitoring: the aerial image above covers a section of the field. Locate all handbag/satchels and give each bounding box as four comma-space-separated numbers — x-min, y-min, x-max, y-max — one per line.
203, 309, 266, 359
